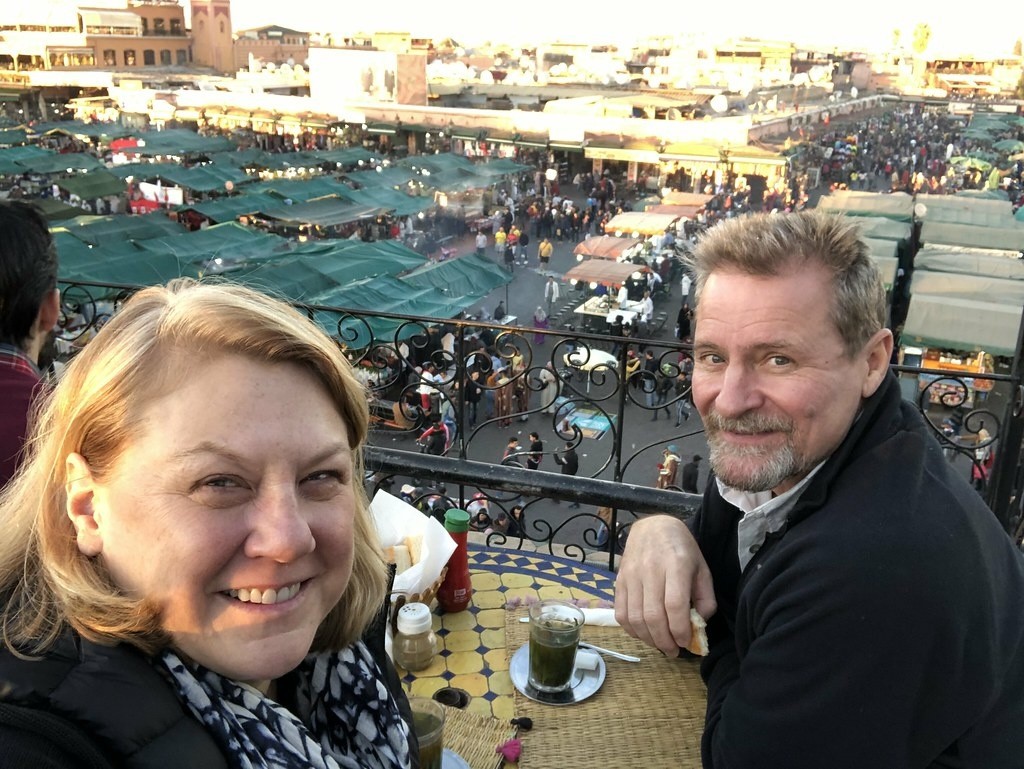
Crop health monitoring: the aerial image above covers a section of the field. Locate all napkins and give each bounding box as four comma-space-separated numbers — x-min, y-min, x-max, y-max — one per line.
541, 605, 620, 627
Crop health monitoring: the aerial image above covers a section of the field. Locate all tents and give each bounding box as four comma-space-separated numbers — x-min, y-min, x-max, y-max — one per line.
0, 117, 531, 236
951, 95, 1022, 171
605, 212, 680, 238
573, 233, 646, 262
813, 188, 1024, 361
47, 203, 513, 411
567, 259, 648, 305
661, 190, 720, 208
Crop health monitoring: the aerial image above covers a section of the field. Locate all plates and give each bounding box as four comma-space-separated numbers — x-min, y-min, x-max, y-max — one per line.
509, 642, 607, 705
442, 748, 471, 769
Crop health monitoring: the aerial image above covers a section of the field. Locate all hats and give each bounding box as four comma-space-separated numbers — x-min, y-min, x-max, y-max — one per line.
668, 445, 678, 452
694, 454, 703, 461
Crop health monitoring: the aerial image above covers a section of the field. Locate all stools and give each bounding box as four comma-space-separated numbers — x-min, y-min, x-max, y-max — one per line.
549, 284, 668, 350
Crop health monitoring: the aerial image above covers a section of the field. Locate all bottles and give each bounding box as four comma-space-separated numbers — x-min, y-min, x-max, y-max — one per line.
435, 508, 473, 613
392, 602, 437, 672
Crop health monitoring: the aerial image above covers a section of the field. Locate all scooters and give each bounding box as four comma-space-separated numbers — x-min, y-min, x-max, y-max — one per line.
412, 440, 430, 486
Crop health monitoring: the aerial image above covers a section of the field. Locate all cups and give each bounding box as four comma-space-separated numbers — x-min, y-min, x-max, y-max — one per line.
407, 696, 446, 769
528, 598, 586, 703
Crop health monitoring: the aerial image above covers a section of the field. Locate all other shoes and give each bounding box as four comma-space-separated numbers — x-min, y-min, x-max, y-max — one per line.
685, 413, 691, 420
517, 418, 528, 422
676, 424, 681, 428
652, 418, 657, 421
667, 413, 671, 418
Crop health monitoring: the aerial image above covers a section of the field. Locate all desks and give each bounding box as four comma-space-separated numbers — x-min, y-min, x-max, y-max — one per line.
474, 217, 504, 230
374, 400, 425, 424
573, 296, 621, 327
564, 347, 618, 394
464, 315, 517, 353
394, 545, 707, 769
415, 351, 476, 396
605, 309, 637, 327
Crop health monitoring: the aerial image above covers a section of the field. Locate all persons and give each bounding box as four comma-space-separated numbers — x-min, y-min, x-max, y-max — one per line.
0, 199, 61, 492
361, 157, 805, 303
607, 213, 1024, 769
0, 286, 426, 769
371, 302, 705, 548
912, 352, 996, 489
789, 111, 1024, 213
